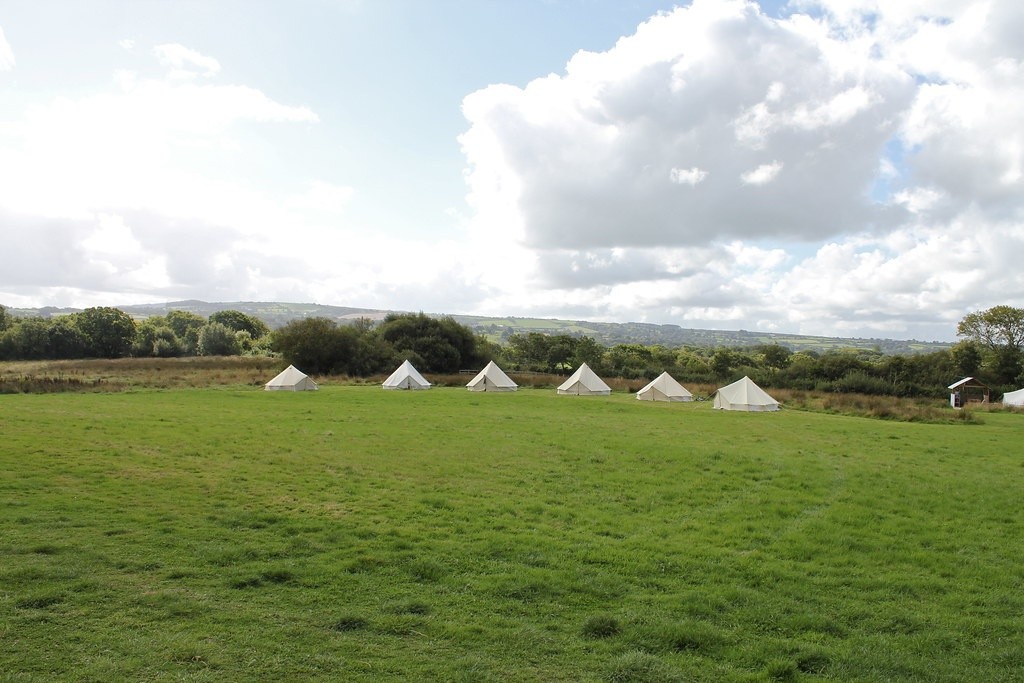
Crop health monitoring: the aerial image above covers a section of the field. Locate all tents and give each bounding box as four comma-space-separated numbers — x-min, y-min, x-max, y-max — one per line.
1001, 387, 1024, 407
711, 375, 782, 412
636, 370, 694, 403
466, 359, 519, 392
264, 363, 319, 392
381, 359, 432, 390
557, 362, 612, 396
